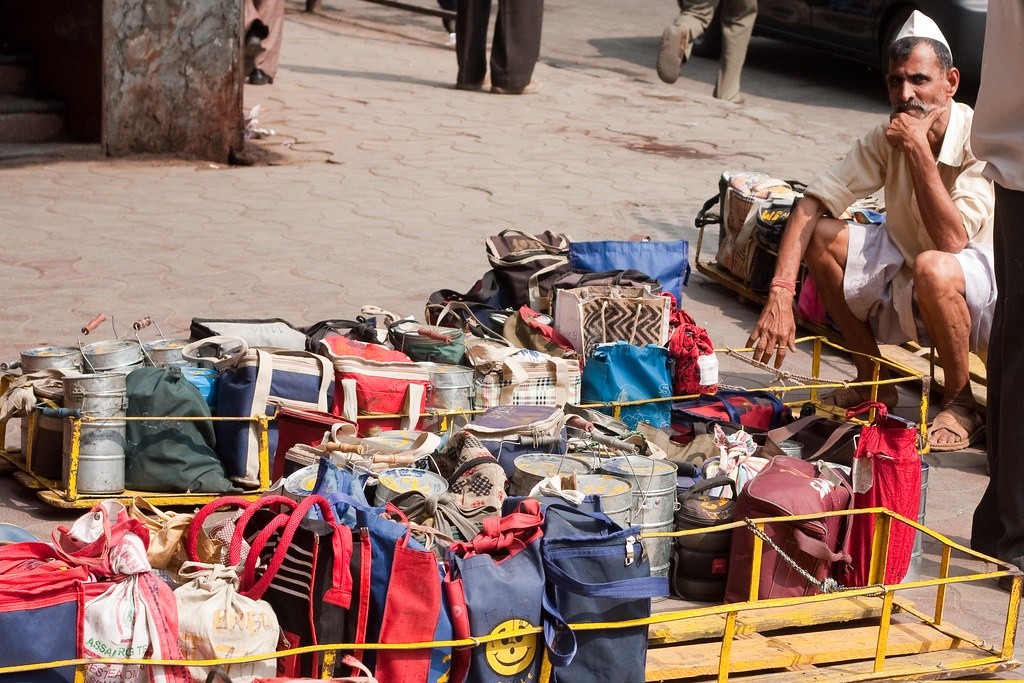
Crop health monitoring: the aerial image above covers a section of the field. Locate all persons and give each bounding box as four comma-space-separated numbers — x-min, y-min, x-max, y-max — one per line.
657, 0, 758, 105
455, 0, 544, 94
744, 10, 998, 451
243, 0, 285, 85
970, 0, 1024, 590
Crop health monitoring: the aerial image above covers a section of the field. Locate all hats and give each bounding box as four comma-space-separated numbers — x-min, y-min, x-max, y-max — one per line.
896, 10, 953, 66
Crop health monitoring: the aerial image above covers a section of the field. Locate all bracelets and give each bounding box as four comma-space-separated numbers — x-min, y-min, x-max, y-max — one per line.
769, 276, 799, 296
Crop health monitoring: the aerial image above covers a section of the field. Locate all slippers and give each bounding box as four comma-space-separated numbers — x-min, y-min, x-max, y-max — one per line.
817, 386, 894, 420
929, 413, 988, 451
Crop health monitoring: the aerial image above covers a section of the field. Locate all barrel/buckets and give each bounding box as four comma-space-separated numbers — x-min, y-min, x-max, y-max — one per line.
755, 435, 804, 457
133, 316, 199, 367
375, 468, 448, 508
78, 314, 145, 376
853, 432, 930, 582
598, 439, 681, 603
20, 347, 85, 429
508, 439, 596, 497
398, 327, 476, 436
62, 372, 127, 494
175, 367, 220, 415
283, 464, 335, 535
567, 450, 633, 530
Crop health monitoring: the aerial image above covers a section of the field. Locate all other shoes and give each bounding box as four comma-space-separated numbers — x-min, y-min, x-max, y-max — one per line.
734, 94, 745, 104
658, 25, 686, 84
249, 69, 269, 84
491, 81, 541, 94
457, 74, 494, 90
244, 32, 261, 75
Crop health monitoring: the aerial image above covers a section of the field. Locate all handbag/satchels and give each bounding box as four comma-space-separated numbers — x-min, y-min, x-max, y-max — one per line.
695, 170, 815, 291
0, 228, 922, 683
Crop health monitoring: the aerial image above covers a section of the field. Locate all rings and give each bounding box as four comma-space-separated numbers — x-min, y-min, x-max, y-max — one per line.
778, 345, 787, 349
